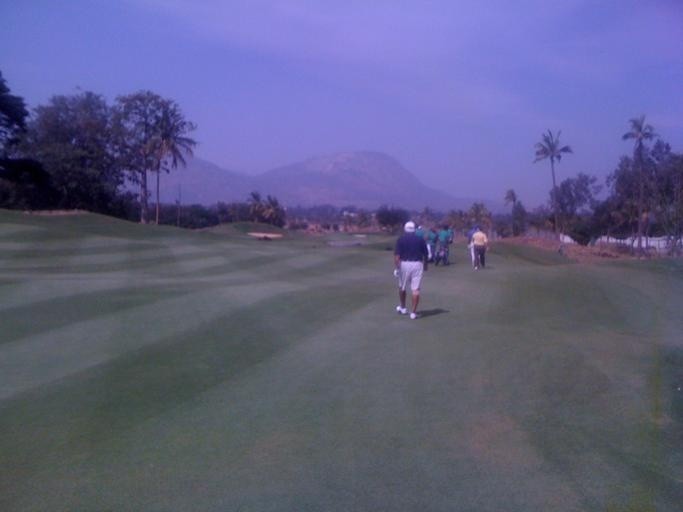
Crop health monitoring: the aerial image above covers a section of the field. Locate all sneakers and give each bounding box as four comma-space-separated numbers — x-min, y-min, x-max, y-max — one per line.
410, 312, 417, 320
396, 305, 409, 315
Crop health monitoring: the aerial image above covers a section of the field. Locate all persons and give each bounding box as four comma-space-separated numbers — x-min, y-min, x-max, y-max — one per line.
392, 221, 429, 320
415, 223, 487, 271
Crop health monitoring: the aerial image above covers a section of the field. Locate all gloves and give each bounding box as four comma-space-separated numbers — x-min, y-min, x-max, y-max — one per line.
393, 268, 400, 278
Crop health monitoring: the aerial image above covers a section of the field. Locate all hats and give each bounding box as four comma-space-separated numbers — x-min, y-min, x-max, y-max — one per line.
404, 221, 416, 233
